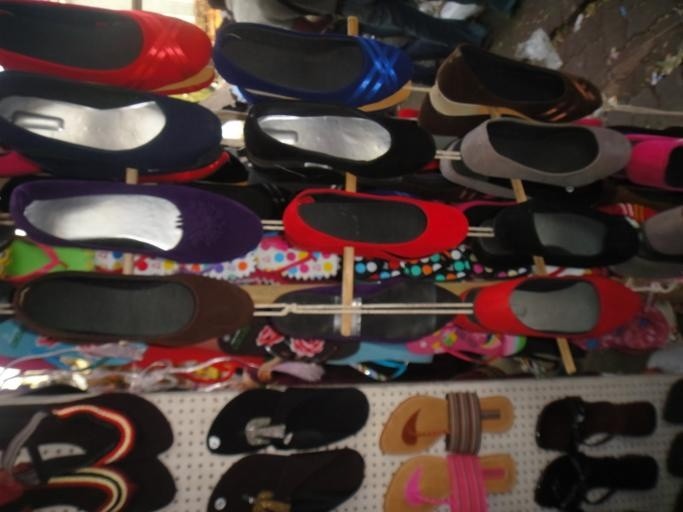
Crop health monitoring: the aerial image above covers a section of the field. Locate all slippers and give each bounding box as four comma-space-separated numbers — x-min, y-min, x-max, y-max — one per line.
1, 387, 658, 512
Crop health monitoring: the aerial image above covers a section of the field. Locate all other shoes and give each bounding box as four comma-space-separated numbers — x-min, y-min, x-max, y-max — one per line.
0, 0, 683, 348
